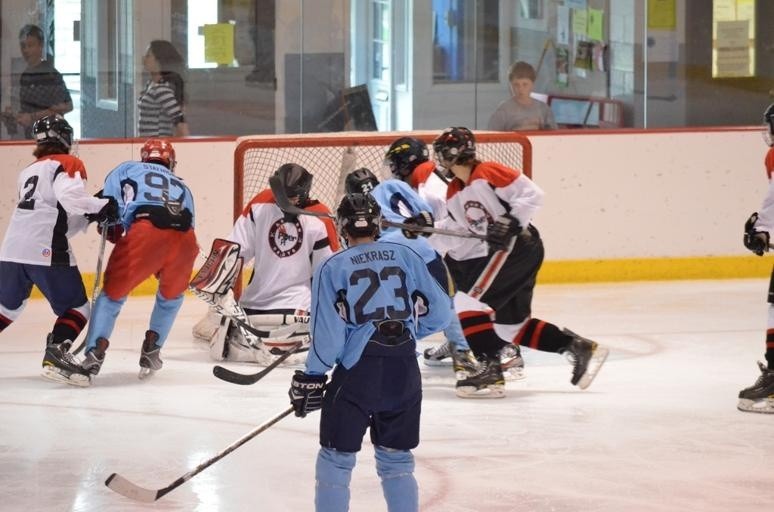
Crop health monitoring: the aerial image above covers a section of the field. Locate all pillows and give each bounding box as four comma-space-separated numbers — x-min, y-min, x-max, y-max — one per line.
273, 127, 476, 245
33, 115, 73, 148
140, 137, 177, 172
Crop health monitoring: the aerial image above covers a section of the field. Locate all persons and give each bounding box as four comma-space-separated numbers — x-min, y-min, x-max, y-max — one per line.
737, 111, 774, 414
80, 138, 199, 381
136, 40, 190, 139
2, 25, 72, 137
1, 112, 120, 388
289, 194, 454, 512
190, 164, 346, 363
487, 61, 558, 131
383, 139, 526, 382
403, 126, 609, 399
344, 167, 482, 380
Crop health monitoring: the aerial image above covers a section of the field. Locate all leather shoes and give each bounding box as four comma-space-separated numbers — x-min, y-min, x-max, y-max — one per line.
288, 370, 328, 418
487, 213, 523, 250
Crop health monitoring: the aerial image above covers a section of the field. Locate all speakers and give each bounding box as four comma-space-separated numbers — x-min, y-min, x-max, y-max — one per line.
424, 327, 597, 389
739, 362, 774, 398
43, 330, 162, 375
